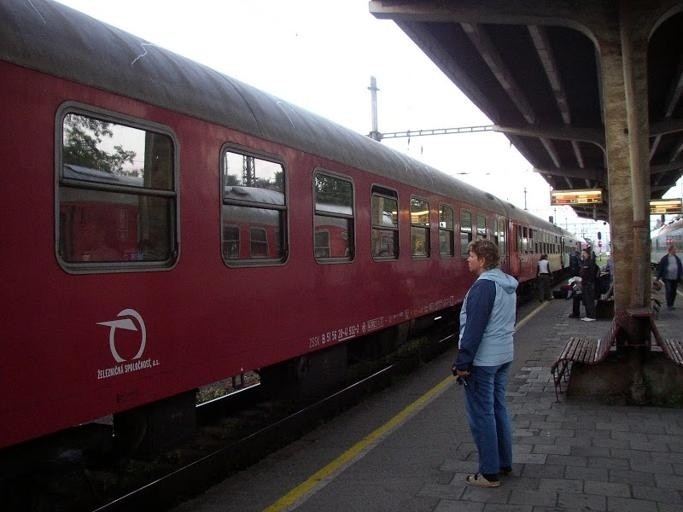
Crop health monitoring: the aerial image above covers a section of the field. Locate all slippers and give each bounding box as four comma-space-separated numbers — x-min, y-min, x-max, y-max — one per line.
465, 472, 501, 488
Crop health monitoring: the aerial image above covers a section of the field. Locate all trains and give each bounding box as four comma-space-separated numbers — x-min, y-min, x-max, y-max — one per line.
650, 215, 683, 269
1, 0, 593, 455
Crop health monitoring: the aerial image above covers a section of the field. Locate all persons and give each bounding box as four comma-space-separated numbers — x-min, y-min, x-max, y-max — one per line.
556, 246, 612, 322
449, 239, 520, 486
535, 254, 556, 302
656, 244, 682, 310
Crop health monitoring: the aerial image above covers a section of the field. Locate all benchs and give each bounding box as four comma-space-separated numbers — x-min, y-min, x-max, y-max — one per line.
594, 282, 612, 319
649, 319, 681, 367
551, 317, 618, 399
